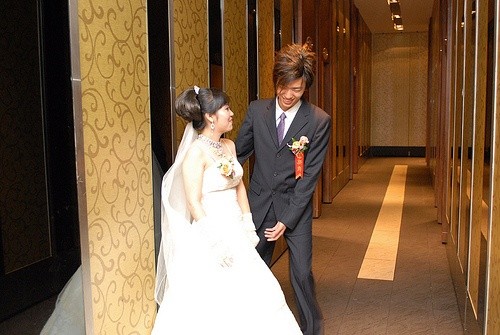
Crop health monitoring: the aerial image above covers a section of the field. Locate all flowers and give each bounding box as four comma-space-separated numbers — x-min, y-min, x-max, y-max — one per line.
217, 155, 234, 178
286, 136, 309, 155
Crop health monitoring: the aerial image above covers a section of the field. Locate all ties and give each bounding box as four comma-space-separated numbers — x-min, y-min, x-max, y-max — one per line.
276, 113, 286, 146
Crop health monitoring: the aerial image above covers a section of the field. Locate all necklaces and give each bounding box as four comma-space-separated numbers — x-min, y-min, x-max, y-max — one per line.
198, 133, 226, 158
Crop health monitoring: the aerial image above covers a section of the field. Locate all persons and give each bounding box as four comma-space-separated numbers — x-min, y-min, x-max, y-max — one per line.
232, 38, 331, 335
150, 84, 306, 335
